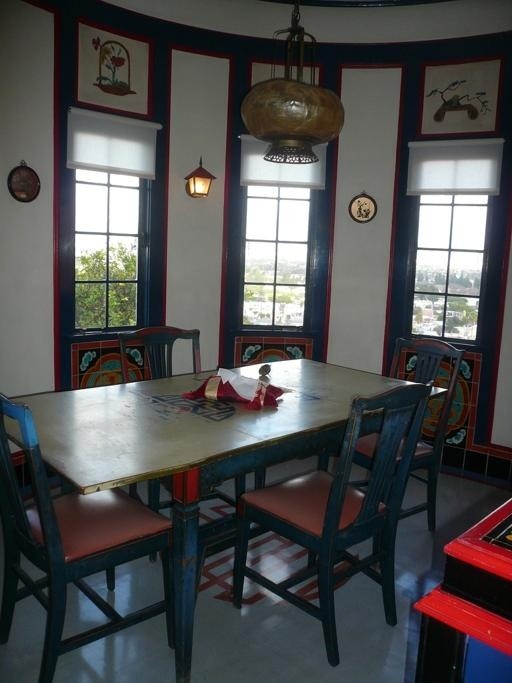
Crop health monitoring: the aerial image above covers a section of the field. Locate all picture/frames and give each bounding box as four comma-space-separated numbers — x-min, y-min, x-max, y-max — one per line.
348, 191, 377, 223
4, 160, 40, 204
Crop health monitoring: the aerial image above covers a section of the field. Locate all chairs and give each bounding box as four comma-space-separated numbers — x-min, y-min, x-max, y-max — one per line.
233, 380, 436, 668
342, 336, 467, 533
116, 324, 236, 566
1, 398, 176, 682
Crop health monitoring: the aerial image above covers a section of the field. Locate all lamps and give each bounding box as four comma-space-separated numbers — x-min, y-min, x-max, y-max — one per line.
182, 157, 217, 198
240, 3, 345, 166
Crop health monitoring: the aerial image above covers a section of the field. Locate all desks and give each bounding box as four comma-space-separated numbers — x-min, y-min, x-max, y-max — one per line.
3, 357, 446, 682
